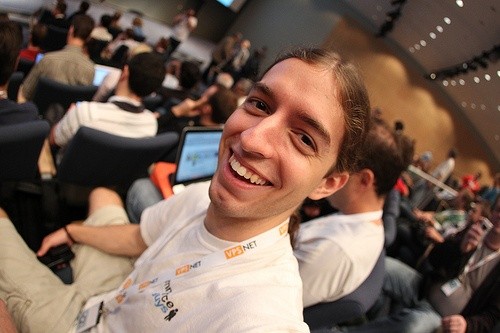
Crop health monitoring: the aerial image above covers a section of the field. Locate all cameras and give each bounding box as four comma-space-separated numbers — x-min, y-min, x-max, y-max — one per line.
37, 243, 74, 284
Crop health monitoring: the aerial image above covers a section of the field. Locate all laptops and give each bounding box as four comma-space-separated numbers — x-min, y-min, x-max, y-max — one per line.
35, 52, 44, 64
171, 125, 224, 196
93, 63, 122, 87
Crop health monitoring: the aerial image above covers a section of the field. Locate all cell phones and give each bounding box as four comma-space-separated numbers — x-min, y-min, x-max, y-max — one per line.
480, 218, 494, 233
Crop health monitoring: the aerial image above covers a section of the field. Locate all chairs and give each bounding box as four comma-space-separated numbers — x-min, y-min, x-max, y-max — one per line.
0, 25, 204, 239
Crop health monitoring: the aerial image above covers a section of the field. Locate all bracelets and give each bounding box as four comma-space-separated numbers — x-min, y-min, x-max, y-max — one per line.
63, 225, 77, 244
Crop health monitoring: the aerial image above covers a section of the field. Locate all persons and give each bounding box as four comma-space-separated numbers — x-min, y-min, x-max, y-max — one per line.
0, 0, 500, 258
348, 196, 500, 333
125, 116, 406, 308
0, 46, 371, 333
